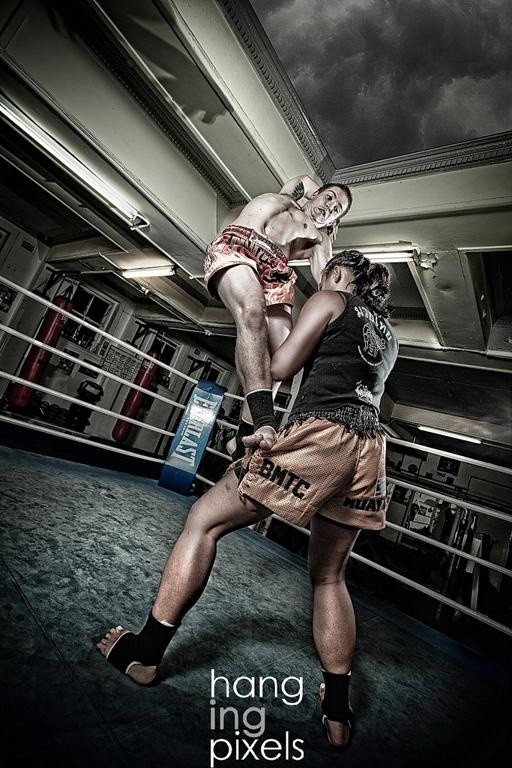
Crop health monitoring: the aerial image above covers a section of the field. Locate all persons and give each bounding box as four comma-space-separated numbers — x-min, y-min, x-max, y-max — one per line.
205, 174, 354, 464
92, 251, 399, 747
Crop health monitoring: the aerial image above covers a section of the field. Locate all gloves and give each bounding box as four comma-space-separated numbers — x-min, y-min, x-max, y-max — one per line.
260, 266, 298, 316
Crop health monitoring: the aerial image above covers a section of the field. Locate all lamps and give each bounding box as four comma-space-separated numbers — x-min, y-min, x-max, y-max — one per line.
0, 94, 138, 222
121, 264, 178, 278
416, 424, 483, 445
